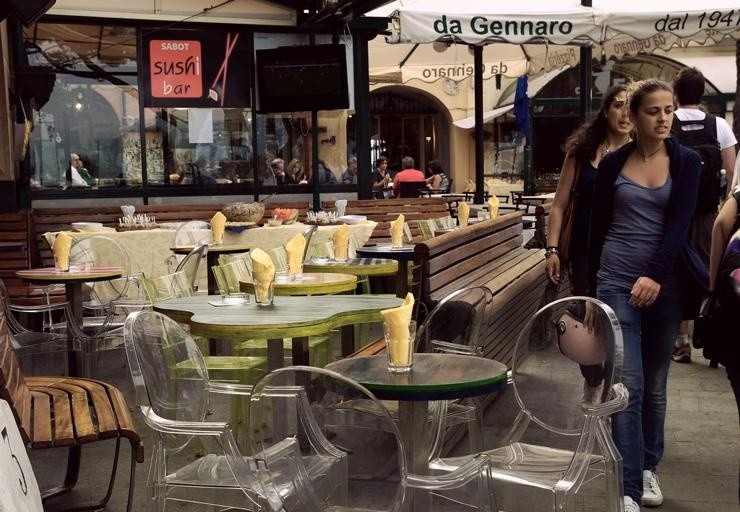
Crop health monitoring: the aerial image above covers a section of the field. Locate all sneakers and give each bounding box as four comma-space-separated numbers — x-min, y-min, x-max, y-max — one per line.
623, 496, 640, 512
671, 342, 691, 362
582, 378, 606, 411
641, 470, 663, 506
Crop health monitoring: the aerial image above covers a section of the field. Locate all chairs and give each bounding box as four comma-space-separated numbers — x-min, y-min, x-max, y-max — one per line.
390, 181, 429, 198
430, 179, 452, 194
430, 296, 629, 512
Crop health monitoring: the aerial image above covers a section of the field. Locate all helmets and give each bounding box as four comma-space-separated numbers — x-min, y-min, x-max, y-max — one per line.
548, 302, 607, 365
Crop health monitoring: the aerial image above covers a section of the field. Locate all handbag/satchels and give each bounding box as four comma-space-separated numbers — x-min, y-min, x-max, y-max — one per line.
558, 200, 576, 266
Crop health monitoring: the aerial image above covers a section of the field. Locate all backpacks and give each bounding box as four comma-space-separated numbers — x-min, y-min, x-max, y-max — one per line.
671, 111, 722, 215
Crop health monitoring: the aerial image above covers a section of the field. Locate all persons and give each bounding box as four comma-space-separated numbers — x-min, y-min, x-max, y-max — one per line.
62, 153, 89, 186
163, 145, 450, 200
578, 77, 703, 512
77, 162, 97, 186
544, 68, 740, 416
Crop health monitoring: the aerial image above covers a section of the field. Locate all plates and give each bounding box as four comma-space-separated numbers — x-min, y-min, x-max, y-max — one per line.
340, 214, 367, 224
71, 222, 102, 232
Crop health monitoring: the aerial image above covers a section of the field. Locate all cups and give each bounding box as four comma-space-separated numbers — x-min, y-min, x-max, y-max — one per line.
381, 317, 419, 373
211, 222, 228, 245
389, 227, 407, 250
487, 203, 501, 220
457, 212, 471, 228
283, 243, 308, 281
51, 247, 71, 272
332, 238, 349, 262
251, 265, 278, 306
